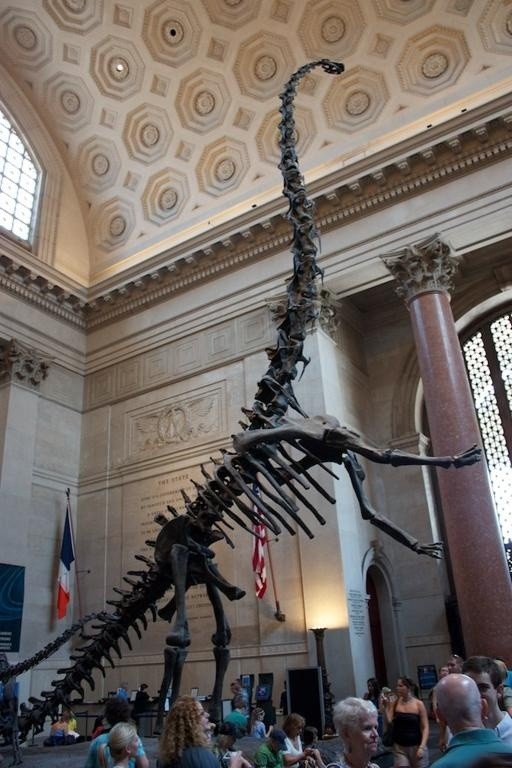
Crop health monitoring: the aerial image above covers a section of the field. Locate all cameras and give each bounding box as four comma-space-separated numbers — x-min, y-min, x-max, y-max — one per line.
223, 751, 237, 760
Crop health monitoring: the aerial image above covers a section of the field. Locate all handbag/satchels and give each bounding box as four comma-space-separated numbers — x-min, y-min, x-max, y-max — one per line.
383, 720, 394, 747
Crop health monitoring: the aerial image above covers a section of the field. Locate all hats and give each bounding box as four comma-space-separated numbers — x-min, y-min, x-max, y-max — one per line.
219, 722, 243, 738
270, 730, 288, 750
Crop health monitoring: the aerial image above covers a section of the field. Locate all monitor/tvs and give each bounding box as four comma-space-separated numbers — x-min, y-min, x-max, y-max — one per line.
256, 684, 271, 700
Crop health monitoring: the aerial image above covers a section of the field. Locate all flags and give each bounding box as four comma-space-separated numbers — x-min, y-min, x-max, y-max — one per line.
250, 479, 269, 599
57, 501, 75, 619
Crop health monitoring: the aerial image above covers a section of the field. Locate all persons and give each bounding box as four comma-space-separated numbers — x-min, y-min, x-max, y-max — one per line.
429, 655, 511, 767
322, 676, 429, 767
86, 681, 155, 766
53, 709, 80, 746
156, 679, 326, 768
